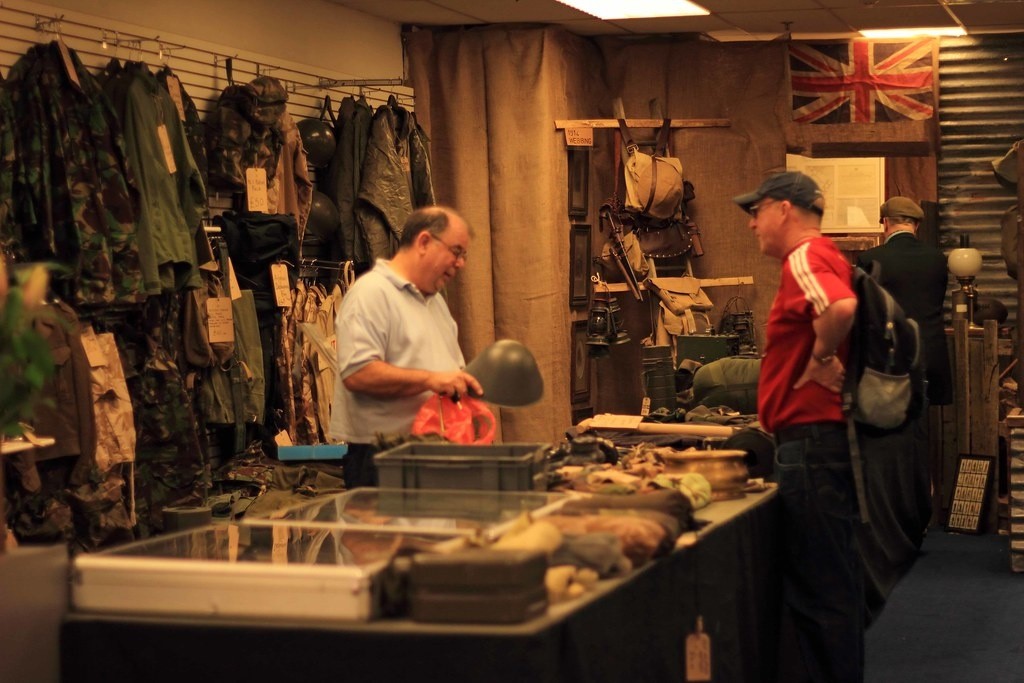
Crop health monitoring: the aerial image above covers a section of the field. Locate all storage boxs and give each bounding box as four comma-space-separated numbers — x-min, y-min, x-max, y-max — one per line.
70, 441, 558, 626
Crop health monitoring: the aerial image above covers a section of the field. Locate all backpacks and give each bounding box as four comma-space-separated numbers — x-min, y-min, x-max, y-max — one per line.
840, 265, 921, 431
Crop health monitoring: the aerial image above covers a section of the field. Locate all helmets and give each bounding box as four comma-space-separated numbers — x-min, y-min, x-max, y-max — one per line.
462, 340, 545, 407
296, 119, 336, 165
308, 191, 339, 243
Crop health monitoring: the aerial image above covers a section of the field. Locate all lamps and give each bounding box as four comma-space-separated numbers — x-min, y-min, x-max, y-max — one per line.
947, 234, 983, 324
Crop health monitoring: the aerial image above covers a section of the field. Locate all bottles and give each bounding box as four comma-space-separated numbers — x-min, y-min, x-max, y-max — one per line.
883, 348, 898, 376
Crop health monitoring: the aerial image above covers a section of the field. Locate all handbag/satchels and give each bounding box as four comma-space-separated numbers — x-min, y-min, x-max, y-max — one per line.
603, 119, 755, 349
198, 244, 265, 428
672, 352, 762, 414
183, 225, 235, 368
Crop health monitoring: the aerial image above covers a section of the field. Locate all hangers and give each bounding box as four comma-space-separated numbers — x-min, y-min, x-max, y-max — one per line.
38, 18, 67, 45
110, 39, 148, 76
299, 259, 348, 288
159, 47, 174, 75
351, 79, 404, 111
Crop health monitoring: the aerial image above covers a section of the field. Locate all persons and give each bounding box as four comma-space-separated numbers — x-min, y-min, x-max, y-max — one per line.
327, 206, 484, 491
733, 171, 866, 683
856, 196, 954, 519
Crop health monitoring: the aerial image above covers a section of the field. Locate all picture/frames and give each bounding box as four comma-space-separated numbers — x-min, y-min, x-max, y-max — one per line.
568, 147, 593, 425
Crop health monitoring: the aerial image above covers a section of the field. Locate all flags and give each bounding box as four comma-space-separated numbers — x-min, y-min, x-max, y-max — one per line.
788, 38, 934, 123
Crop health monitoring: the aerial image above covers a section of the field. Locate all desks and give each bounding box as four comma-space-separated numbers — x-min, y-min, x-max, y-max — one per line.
57, 480, 778, 683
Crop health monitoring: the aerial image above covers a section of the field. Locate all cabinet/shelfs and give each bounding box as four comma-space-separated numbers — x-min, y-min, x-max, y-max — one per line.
940, 315, 1024, 576
555, 119, 754, 290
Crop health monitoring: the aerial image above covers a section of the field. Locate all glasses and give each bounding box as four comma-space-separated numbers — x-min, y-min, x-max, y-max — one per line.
750, 200, 775, 218
428, 233, 467, 262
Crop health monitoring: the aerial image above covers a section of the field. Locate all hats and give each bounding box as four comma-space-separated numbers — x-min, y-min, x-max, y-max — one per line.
879, 196, 924, 224
244, 77, 288, 125
733, 171, 824, 216
